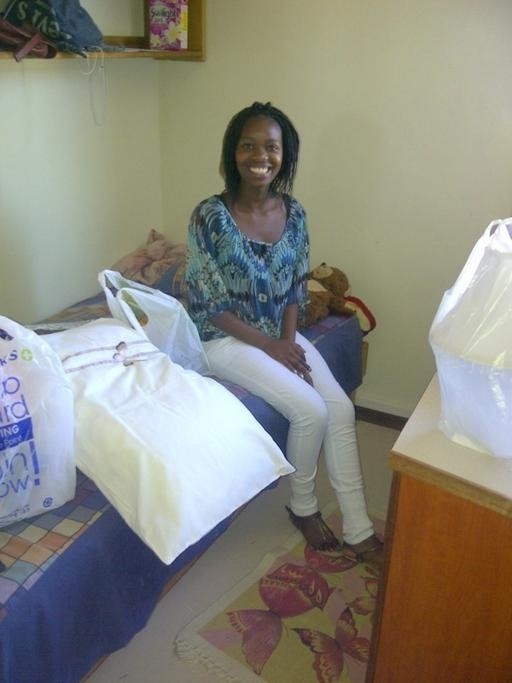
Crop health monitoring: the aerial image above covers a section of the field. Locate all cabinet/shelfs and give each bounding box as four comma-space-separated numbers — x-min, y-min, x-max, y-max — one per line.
364, 372, 512, 683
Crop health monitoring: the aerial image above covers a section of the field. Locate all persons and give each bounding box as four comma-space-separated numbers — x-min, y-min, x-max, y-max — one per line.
187, 101, 387, 575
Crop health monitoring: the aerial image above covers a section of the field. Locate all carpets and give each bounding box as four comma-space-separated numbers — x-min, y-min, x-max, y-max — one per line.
174, 495, 385, 683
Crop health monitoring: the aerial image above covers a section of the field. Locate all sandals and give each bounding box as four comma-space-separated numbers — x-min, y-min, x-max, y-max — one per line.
286, 505, 344, 558
343, 536, 386, 576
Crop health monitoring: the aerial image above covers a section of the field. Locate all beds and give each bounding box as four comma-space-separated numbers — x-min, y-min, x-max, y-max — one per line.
0, 229, 369, 683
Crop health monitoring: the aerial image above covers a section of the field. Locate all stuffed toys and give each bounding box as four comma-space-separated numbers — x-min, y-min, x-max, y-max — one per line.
296, 262, 357, 332
